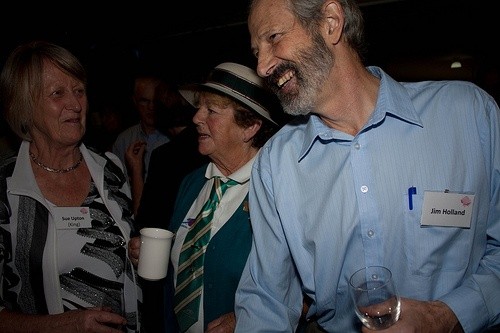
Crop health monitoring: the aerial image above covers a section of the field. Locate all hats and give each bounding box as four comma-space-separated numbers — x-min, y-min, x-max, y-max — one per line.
178, 62, 281, 132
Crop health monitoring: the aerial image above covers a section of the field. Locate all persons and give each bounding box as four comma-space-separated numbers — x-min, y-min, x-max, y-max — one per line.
233, 0, 500, 333
0, 42, 142, 333
84, 48, 217, 333
128, 62, 313, 333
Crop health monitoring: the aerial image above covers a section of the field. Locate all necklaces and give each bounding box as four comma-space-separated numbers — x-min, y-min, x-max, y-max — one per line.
28, 150, 83, 175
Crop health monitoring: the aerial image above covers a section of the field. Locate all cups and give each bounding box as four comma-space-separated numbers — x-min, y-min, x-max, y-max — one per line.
348, 266, 401, 330
137, 228, 174, 281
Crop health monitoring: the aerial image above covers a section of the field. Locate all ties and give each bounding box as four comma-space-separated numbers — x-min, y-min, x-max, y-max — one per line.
172, 177, 238, 333
